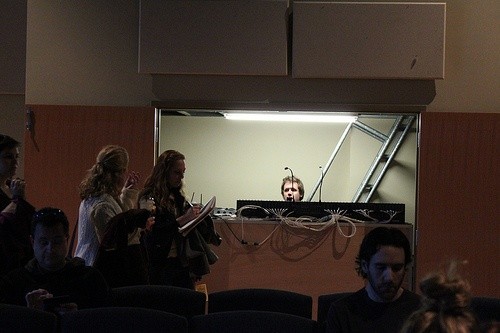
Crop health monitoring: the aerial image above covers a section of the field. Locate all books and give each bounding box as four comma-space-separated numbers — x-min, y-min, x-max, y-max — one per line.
178, 196, 217, 237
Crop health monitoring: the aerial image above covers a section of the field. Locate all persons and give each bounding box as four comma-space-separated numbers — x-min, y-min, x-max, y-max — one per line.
281, 176, 304, 202
0, 133, 38, 281
24, 204, 112, 315
77, 144, 157, 284
70, 180, 128, 265
323, 227, 424, 333
401, 271, 478, 333
135, 149, 202, 284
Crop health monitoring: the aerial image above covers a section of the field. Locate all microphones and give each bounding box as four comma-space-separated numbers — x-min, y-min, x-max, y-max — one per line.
285, 167, 293, 201
319, 166, 323, 202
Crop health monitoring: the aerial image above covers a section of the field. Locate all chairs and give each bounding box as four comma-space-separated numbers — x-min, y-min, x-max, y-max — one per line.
0, 284, 500, 333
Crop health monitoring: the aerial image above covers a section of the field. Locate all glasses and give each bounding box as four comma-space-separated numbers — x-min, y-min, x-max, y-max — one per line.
32, 209, 65, 232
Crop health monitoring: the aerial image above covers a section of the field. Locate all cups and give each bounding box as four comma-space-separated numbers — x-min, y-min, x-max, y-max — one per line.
138, 198, 154, 222
